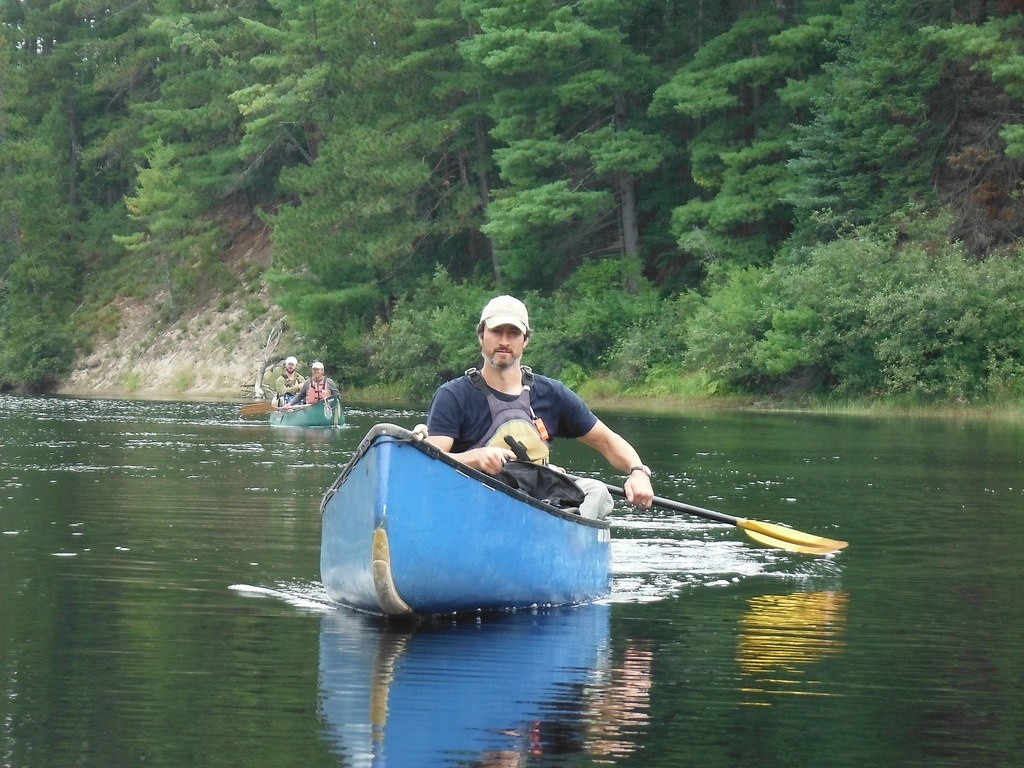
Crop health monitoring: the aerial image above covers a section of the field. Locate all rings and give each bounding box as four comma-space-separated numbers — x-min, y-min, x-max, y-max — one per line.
642, 502, 646, 506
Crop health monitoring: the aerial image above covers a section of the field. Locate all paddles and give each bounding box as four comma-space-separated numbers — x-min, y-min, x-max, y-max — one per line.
242, 403, 312, 416
562, 471, 851, 556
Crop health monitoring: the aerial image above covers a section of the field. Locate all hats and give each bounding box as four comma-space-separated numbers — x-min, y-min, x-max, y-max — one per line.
479, 295, 529, 335
312, 362, 324, 369
285, 356, 298, 365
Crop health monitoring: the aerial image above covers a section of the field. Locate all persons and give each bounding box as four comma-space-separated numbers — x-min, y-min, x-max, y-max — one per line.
426, 294, 654, 517
282, 362, 341, 409
275, 356, 307, 405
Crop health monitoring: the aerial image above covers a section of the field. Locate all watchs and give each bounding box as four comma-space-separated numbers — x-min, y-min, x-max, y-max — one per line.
630, 465, 651, 477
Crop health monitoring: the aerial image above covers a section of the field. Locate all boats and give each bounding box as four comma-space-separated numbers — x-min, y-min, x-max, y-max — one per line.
270, 395, 345, 426
317, 604, 610, 768
320, 423, 611, 618
